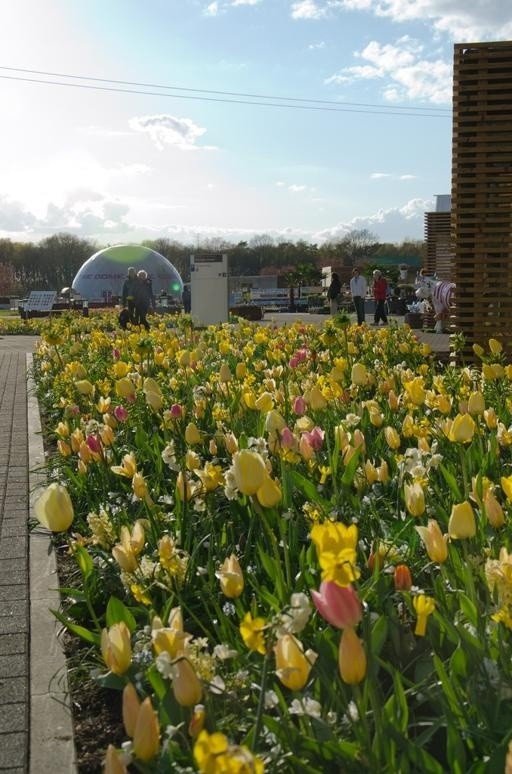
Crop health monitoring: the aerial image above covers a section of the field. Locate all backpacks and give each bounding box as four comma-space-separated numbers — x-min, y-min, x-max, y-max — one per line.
119, 309, 138, 331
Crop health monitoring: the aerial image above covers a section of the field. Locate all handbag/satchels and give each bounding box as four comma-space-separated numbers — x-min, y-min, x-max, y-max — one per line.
336, 294, 344, 302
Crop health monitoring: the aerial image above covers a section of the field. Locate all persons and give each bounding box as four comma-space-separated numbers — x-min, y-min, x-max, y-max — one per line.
181, 284, 192, 313
349, 267, 368, 327
327, 271, 342, 315
132, 269, 156, 330
369, 269, 390, 326
120, 265, 151, 327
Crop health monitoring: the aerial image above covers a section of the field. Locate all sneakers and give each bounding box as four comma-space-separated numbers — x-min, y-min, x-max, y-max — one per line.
380, 322, 388, 326
370, 321, 378, 326
